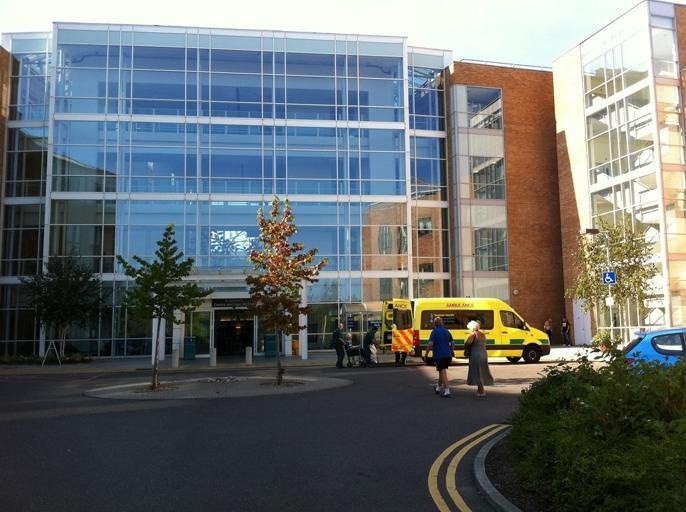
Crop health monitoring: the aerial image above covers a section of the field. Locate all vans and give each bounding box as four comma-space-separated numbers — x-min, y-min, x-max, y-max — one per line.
378, 296, 551, 366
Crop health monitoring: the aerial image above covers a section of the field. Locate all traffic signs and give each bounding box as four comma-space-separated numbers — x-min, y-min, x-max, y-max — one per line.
579, 227, 616, 347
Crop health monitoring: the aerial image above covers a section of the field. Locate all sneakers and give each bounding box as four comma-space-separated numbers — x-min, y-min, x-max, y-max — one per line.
433, 386, 452, 398
473, 392, 488, 400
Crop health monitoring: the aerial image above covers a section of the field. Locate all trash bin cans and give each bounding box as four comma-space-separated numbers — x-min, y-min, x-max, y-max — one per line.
184, 336, 196, 360
264, 333, 278, 357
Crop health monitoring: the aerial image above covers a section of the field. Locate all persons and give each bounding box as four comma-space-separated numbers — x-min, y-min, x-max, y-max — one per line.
560, 315, 572, 346
332, 323, 347, 367
463, 320, 495, 396
363, 325, 378, 368
424, 317, 455, 397
393, 350, 408, 367
543, 319, 553, 347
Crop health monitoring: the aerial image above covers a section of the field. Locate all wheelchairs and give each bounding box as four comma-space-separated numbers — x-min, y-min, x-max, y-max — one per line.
344, 344, 367, 368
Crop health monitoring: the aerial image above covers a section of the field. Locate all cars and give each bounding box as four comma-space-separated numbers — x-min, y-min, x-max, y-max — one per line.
621, 327, 686, 376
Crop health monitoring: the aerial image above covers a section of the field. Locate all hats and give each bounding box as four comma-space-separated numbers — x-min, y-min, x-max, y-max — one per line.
467, 320, 480, 332
